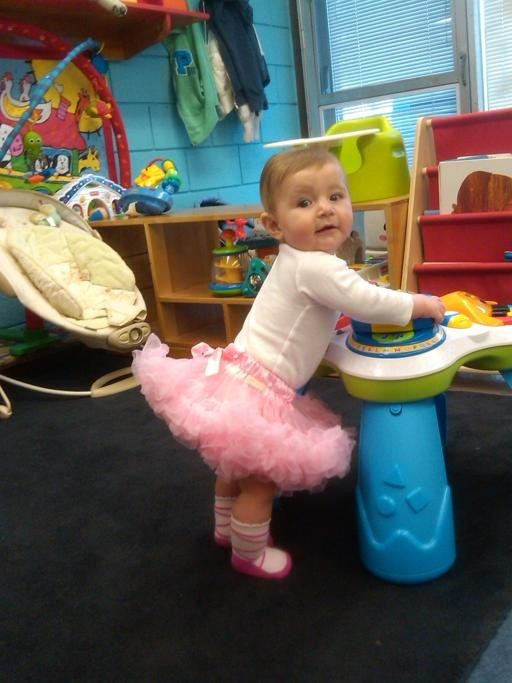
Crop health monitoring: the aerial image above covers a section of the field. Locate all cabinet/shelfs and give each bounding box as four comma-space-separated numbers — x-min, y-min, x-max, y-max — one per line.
88, 185, 410, 359
402, 106, 512, 303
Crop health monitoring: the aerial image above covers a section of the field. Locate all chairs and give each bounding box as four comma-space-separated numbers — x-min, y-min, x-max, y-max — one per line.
0, 190, 152, 419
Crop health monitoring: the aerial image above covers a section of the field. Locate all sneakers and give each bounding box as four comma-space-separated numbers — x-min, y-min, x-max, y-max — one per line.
213, 530, 294, 580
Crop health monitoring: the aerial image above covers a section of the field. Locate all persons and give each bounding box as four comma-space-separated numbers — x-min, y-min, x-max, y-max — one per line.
130, 138, 451, 583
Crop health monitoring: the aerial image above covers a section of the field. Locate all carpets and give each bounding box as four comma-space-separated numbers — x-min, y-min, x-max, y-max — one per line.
1, 341, 511, 682
